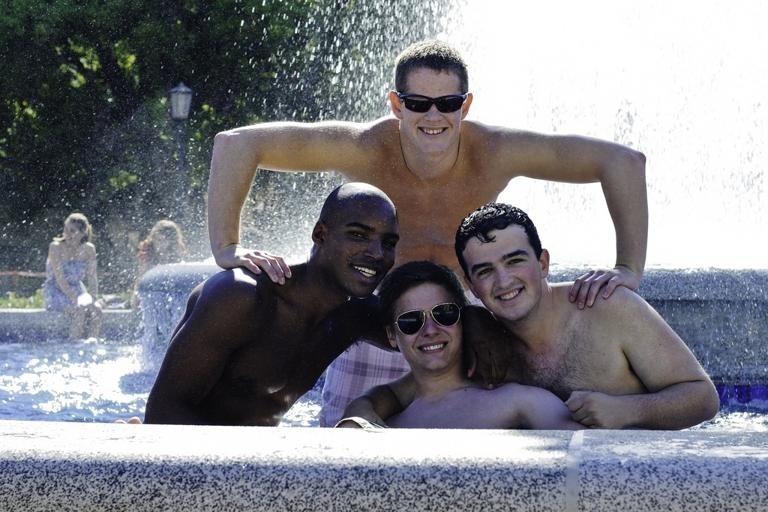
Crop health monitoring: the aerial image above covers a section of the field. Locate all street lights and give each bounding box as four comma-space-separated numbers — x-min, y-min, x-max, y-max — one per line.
169, 79, 194, 225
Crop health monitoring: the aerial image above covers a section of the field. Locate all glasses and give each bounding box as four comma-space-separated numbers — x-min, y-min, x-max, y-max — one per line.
395, 91, 469, 112
394, 302, 462, 336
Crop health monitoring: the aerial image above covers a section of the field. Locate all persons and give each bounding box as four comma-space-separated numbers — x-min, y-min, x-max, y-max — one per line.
142, 182, 503, 426
339, 201, 720, 430
130, 219, 187, 313
206, 40, 649, 309
44, 213, 101, 339
381, 260, 590, 430
